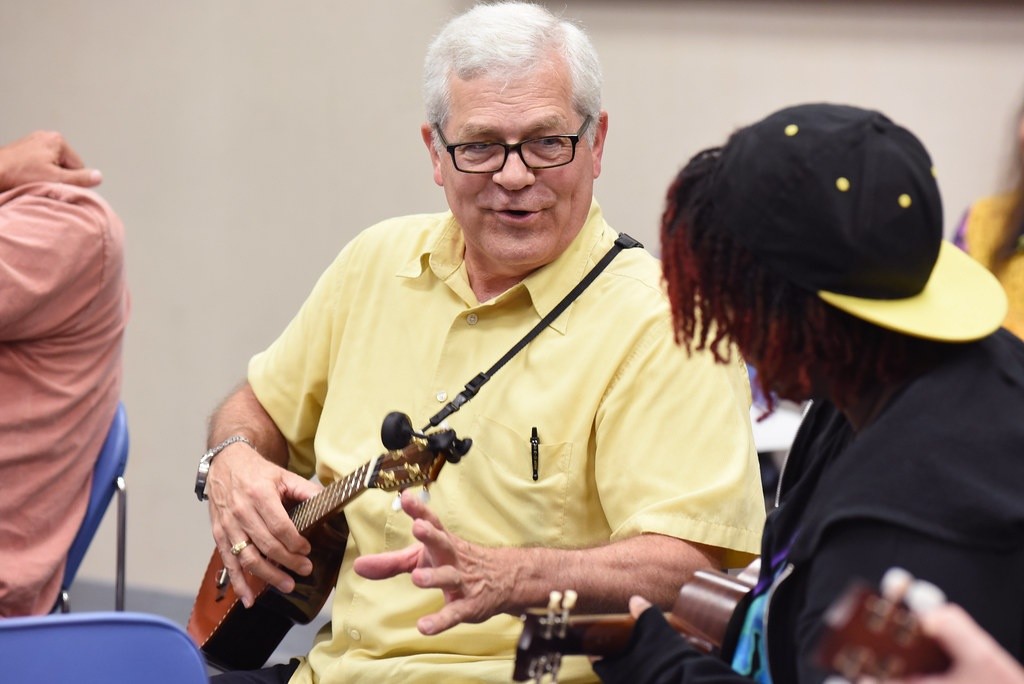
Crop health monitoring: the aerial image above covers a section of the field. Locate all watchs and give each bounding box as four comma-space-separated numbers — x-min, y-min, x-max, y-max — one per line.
194, 435, 258, 503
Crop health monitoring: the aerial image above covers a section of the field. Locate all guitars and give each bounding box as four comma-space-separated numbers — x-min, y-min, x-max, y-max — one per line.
183, 410, 474, 675
811, 561, 956, 684
512, 550, 765, 684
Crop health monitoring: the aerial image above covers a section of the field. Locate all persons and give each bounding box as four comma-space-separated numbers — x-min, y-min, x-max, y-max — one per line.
203, 0, 768, 684
586, 102, 1024, 684
823, 565, 1024, 684
950, 82, 1024, 343
0, 128, 133, 620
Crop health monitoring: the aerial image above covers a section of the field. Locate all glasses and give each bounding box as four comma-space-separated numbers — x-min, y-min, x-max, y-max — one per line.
435, 115, 592, 174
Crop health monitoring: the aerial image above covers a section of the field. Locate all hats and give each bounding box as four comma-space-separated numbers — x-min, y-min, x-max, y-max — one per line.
722, 104, 1005, 339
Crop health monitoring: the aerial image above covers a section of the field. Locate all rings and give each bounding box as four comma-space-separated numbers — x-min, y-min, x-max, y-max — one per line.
230, 540, 253, 555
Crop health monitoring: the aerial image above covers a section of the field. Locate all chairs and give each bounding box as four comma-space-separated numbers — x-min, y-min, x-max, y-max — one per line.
1, 406, 209, 684
